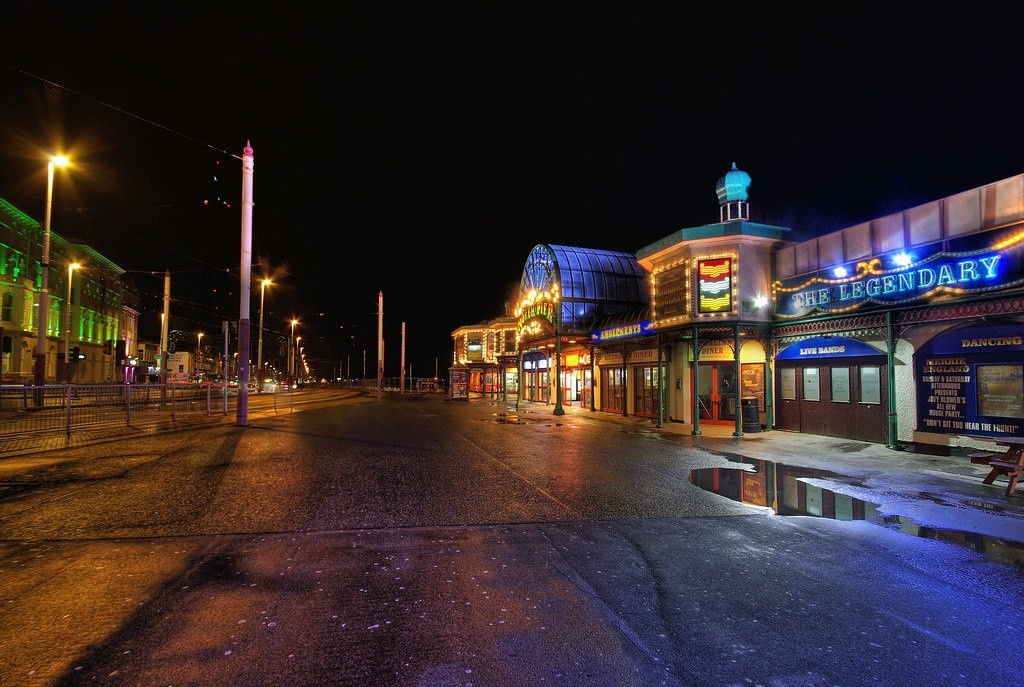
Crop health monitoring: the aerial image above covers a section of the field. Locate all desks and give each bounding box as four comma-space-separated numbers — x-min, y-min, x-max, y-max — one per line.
982, 437, 1024, 497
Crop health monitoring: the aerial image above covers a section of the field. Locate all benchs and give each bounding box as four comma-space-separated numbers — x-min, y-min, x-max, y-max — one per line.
970, 453, 1017, 465
989, 461, 1024, 476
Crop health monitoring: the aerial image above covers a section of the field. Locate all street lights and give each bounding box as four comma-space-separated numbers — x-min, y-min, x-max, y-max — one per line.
296, 336, 305, 378
64, 263, 80, 442
289, 319, 299, 391
32, 153, 70, 409
195, 332, 204, 401
256, 279, 272, 394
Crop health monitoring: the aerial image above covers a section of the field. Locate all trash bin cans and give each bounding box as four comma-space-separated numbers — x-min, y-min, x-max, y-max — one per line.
741, 396, 761, 433
